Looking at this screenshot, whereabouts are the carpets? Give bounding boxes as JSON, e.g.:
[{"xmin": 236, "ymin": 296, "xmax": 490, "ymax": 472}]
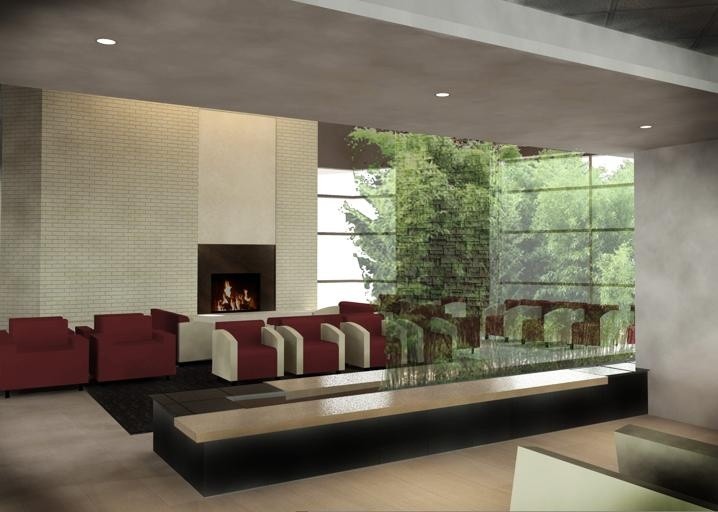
[{"xmin": 86, "ymin": 359, "xmax": 477, "ymax": 435}]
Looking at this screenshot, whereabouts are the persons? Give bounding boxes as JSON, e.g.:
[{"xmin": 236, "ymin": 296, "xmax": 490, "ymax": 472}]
[
  {"xmin": 224, "ymin": 280, "xmax": 240, "ymax": 311},
  {"xmin": 239, "ymin": 289, "xmax": 251, "ymax": 310}
]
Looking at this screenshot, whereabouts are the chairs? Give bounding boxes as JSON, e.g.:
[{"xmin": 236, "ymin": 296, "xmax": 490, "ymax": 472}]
[
  {"xmin": 574, "ymin": 303, "xmax": 620, "ymax": 348},
  {"xmin": 445, "ymin": 297, "xmax": 480, "ymax": 354},
  {"xmin": 617, "ymin": 302, "xmax": 636, "ymax": 346},
  {"xmin": 267, "ymin": 316, "xmax": 347, "ymax": 374},
  {"xmin": 335, "ymin": 313, "xmax": 406, "ymax": 369},
  {"xmin": 211, "ymin": 322, "xmax": 285, "ymax": 381},
  {"xmin": 149, "ymin": 309, "xmax": 215, "ymax": 364},
  {"xmin": 483, "ymin": 296, "xmax": 538, "ymax": 343},
  {"xmin": 0, "ymin": 314, "xmax": 176, "ymax": 399},
  {"xmin": 398, "ymin": 308, "xmax": 455, "ymax": 365},
  {"xmin": 517, "ymin": 297, "xmax": 568, "ymax": 348}
]
